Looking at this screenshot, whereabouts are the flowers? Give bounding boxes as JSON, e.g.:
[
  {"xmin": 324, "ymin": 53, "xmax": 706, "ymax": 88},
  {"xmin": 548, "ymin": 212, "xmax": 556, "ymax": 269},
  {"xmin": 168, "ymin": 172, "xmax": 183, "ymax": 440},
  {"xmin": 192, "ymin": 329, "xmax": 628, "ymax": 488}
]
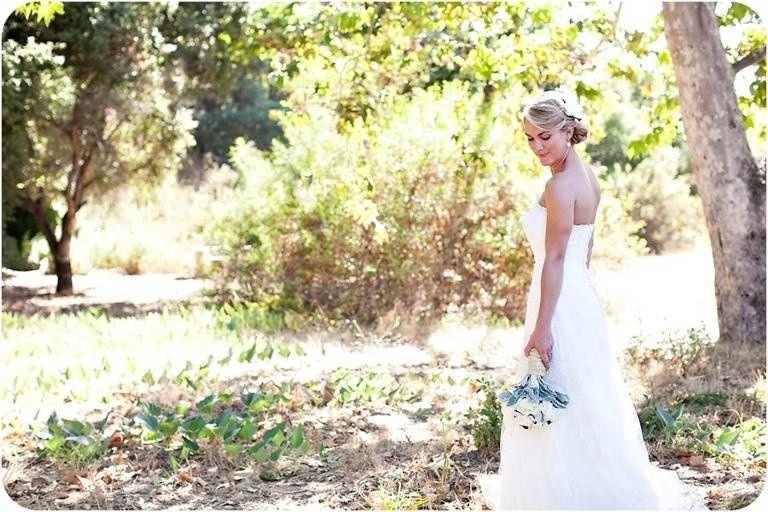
[{"xmin": 497, "ymin": 348, "xmax": 570, "ymax": 433}]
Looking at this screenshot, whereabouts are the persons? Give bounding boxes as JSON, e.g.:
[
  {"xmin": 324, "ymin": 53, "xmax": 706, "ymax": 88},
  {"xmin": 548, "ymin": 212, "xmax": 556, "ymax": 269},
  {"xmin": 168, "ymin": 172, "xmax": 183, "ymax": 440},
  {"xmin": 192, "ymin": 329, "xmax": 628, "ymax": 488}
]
[{"xmin": 475, "ymin": 89, "xmax": 707, "ymax": 512}]
